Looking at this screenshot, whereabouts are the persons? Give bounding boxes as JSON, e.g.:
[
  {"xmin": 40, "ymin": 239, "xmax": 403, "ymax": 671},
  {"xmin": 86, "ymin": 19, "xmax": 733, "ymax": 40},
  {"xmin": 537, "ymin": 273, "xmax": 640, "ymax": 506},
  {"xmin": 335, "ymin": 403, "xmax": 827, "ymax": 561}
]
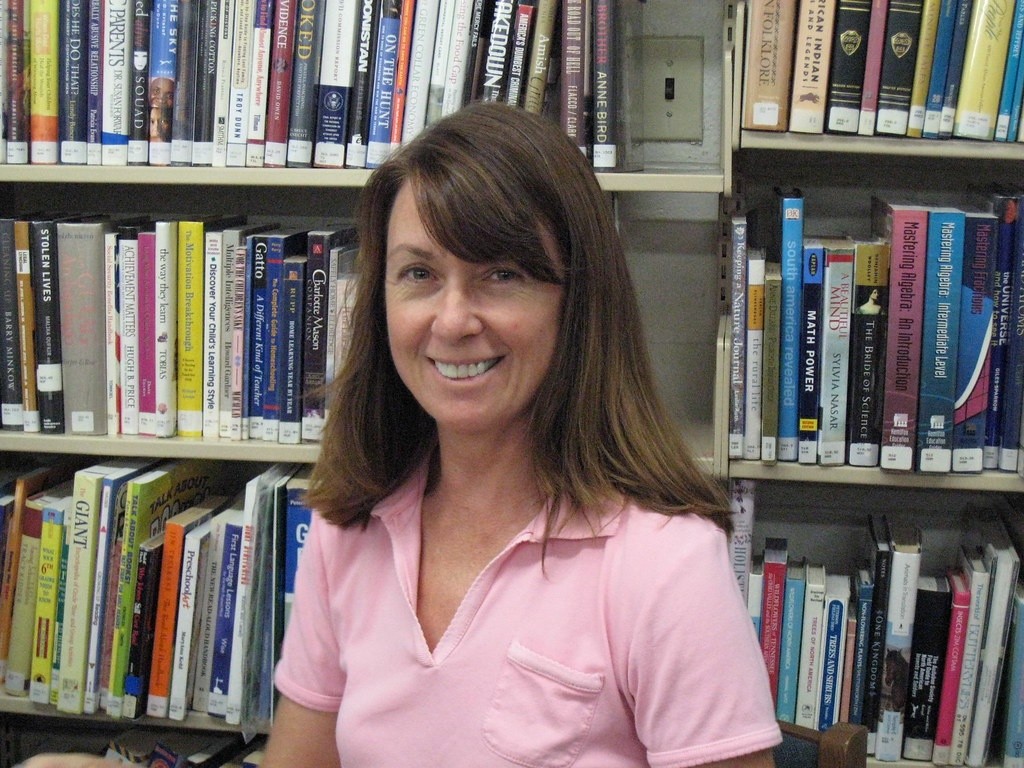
[{"xmin": 257, "ymin": 102, "xmax": 786, "ymax": 767}]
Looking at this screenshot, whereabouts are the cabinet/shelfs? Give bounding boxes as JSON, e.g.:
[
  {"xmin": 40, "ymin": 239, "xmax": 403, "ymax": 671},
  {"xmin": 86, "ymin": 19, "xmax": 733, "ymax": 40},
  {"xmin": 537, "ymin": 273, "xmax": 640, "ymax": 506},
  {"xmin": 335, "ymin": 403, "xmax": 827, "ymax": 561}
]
[
  {"xmin": 721, "ymin": 0, "xmax": 1024, "ymax": 768},
  {"xmin": 0, "ymin": 0, "xmax": 736, "ymax": 768}
]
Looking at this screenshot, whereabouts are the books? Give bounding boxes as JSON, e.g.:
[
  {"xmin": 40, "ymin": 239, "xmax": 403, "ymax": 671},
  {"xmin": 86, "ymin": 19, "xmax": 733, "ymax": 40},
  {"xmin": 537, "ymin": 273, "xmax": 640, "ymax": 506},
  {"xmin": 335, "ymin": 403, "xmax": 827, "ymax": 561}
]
[
  {"xmin": 741, "ymin": 1, "xmax": 1024, "ymax": 143},
  {"xmin": 727, "ymin": 182, "xmax": 1024, "ymax": 479},
  {"xmin": 2, "ymin": 457, "xmax": 315, "ymax": 768},
  {"xmin": 726, "ymin": 476, "xmax": 1023, "ymax": 767},
  {"xmin": 0, "ymin": 209, "xmax": 362, "ymax": 444},
  {"xmin": 1, "ymin": 0, "xmax": 646, "ymax": 174}
]
